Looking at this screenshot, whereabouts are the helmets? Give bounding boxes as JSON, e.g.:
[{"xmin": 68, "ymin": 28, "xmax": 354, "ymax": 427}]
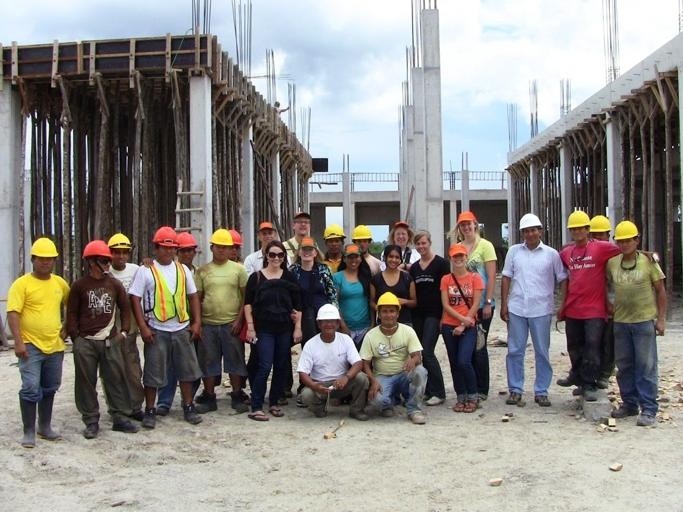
[
  {"xmin": 316, "ymin": 303, "xmax": 342, "ymax": 321},
  {"xmin": 518, "ymin": 210, "xmax": 638, "ymax": 240},
  {"xmin": 29, "ymin": 225, "xmax": 241, "ymax": 258},
  {"xmin": 375, "ymin": 291, "xmax": 402, "ymax": 312}
]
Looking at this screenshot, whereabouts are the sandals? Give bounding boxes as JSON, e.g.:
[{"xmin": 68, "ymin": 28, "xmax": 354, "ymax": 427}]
[{"xmin": 454, "ymin": 397, "xmax": 479, "ymax": 412}]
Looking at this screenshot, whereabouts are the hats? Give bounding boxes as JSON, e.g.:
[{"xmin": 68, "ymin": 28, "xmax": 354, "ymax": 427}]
[{"xmin": 257, "ymin": 211, "xmax": 477, "ymax": 257}]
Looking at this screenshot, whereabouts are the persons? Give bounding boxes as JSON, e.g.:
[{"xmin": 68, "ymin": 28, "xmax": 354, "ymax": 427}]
[
  {"xmin": 439, "ymin": 243, "xmax": 484, "ymax": 413},
  {"xmin": 289, "ymin": 222, "xmax": 451, "ymax": 405},
  {"xmin": 66, "ymin": 227, "xmax": 250, "ymax": 438},
  {"xmin": 7, "ymin": 237, "xmax": 70, "ymax": 448},
  {"xmin": 455, "ymin": 211, "xmax": 498, "ymax": 401},
  {"xmin": 244, "ymin": 239, "xmax": 302, "ymax": 423},
  {"xmin": 297, "ymin": 303, "xmax": 368, "ymax": 422},
  {"xmin": 498, "ymin": 213, "xmax": 569, "ymax": 407},
  {"xmin": 558, "ymin": 211, "xmax": 666, "ymax": 426},
  {"xmin": 359, "ymin": 292, "xmax": 427, "ymax": 423},
  {"xmin": 245, "ymin": 211, "xmax": 321, "ymax": 404}
]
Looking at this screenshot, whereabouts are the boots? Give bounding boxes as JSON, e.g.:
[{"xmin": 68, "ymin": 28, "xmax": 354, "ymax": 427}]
[
  {"xmin": 19, "ymin": 397, "xmax": 36, "ymax": 448},
  {"xmin": 37, "ymin": 392, "xmax": 62, "ymax": 441}
]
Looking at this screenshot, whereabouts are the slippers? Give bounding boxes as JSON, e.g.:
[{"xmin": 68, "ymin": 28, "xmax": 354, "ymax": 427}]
[
  {"xmin": 249, "ymin": 413, "xmax": 269, "ymax": 421},
  {"xmin": 269, "ymin": 409, "xmax": 284, "ymax": 417}
]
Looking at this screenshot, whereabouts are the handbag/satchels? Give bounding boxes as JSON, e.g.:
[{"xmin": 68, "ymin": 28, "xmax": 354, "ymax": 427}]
[
  {"xmin": 239, "ymin": 318, "xmax": 255, "ymax": 343},
  {"xmin": 475, "ymin": 324, "xmax": 487, "ymax": 351}
]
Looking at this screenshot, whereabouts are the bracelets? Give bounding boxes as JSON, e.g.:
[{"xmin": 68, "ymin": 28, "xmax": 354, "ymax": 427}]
[
  {"xmin": 247, "ymin": 329, "xmax": 255, "ymax": 332},
  {"xmin": 247, "ymin": 321, "xmax": 254, "ymax": 325},
  {"xmin": 461, "ymin": 322, "xmax": 465, "ymax": 326}
]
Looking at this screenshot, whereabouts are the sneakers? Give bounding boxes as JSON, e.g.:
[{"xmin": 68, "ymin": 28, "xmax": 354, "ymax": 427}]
[
  {"xmin": 84, "ymin": 390, "xmax": 251, "ymax": 438},
  {"xmin": 507, "ymin": 378, "xmax": 655, "ymax": 426},
  {"xmin": 277, "ymin": 389, "xmax": 445, "ymax": 424}
]
